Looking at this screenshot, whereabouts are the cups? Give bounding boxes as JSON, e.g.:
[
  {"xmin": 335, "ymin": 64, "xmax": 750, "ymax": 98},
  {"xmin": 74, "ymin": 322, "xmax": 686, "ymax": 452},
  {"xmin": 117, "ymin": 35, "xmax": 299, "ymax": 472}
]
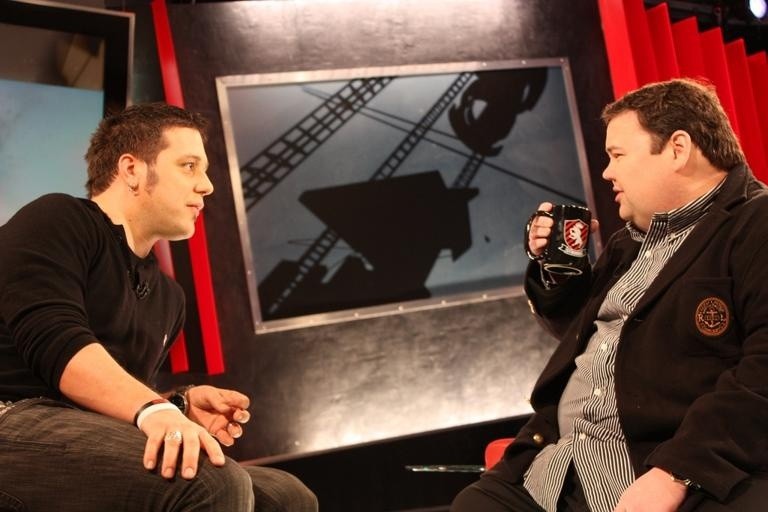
[{"xmin": 523, "ymin": 204, "xmax": 592, "ymax": 277}]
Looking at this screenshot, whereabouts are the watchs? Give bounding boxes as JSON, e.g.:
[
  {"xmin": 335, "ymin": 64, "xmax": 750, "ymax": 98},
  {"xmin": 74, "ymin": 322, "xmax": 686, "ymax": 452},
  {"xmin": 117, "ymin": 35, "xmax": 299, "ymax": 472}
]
[{"xmin": 167, "ymin": 384, "xmax": 195, "ymax": 414}]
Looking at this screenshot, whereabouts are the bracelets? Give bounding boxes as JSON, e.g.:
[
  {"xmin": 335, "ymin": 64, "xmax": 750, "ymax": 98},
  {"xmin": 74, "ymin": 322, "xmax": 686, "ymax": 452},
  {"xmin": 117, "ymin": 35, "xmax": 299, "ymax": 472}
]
[
  {"xmin": 671, "ymin": 472, "xmax": 701, "ymax": 490},
  {"xmin": 133, "ymin": 399, "xmax": 179, "ymax": 430}
]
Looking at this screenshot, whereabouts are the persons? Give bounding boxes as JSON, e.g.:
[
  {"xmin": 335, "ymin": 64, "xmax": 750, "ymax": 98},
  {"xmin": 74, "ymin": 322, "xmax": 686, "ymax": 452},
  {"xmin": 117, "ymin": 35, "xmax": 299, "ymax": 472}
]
[
  {"xmin": 451, "ymin": 79, "xmax": 768, "ymax": 512},
  {"xmin": 0, "ymin": 103, "xmax": 319, "ymax": 512}
]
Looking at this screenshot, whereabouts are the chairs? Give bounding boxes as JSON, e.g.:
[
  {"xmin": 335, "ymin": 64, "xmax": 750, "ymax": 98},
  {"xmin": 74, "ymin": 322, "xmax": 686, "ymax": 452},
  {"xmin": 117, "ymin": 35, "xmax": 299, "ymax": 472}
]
[{"xmin": 406, "ymin": 436, "xmax": 516, "ymax": 477}]
